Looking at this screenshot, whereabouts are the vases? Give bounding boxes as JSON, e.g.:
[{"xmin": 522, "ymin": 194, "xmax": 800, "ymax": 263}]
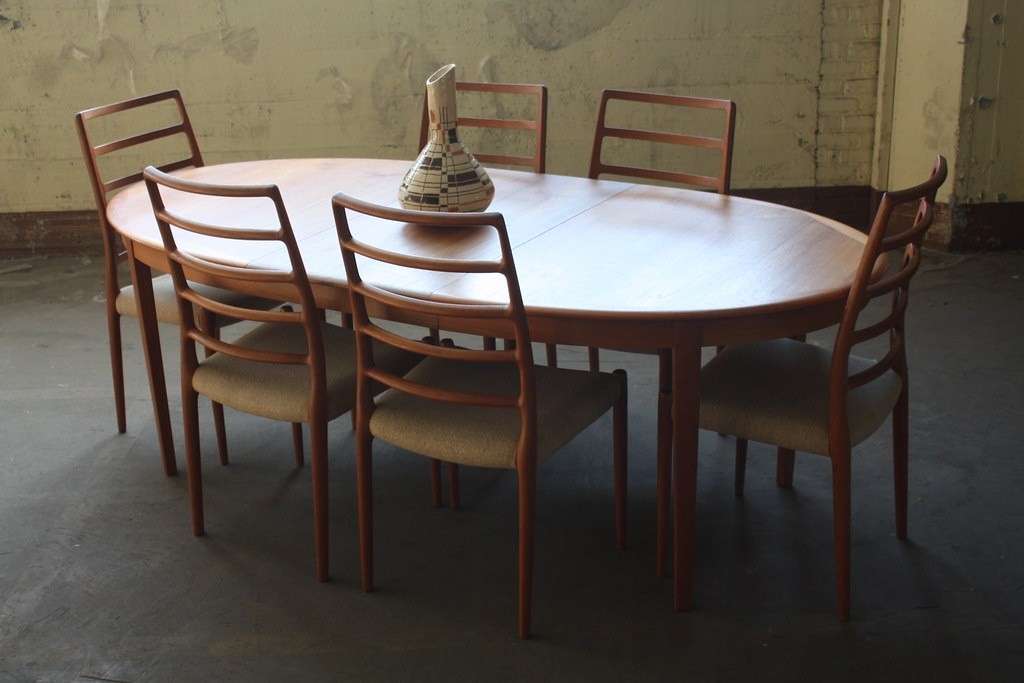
[{"xmin": 399, "ymin": 64, "xmax": 497, "ymax": 213}]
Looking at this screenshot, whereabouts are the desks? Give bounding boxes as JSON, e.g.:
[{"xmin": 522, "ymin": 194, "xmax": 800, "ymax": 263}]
[{"xmin": 106, "ymin": 158, "xmax": 886, "ymax": 616}]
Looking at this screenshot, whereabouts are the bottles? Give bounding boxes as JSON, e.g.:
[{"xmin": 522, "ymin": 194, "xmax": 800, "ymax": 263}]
[{"xmin": 398, "ymin": 64, "xmax": 494, "ymax": 212}]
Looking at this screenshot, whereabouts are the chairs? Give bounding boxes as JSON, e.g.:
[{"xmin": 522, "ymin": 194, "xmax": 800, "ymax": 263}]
[
  {"xmin": 74, "ymin": 89, "xmax": 305, "ymax": 478},
  {"xmin": 418, "ymin": 80, "xmax": 561, "ymax": 365},
  {"xmin": 544, "ymin": 88, "xmax": 737, "ymax": 439},
  {"xmin": 329, "ymin": 190, "xmax": 624, "ymax": 638},
  {"xmin": 695, "ymin": 154, "xmax": 944, "ymax": 615},
  {"xmin": 145, "ymin": 165, "xmax": 439, "ymax": 581}
]
